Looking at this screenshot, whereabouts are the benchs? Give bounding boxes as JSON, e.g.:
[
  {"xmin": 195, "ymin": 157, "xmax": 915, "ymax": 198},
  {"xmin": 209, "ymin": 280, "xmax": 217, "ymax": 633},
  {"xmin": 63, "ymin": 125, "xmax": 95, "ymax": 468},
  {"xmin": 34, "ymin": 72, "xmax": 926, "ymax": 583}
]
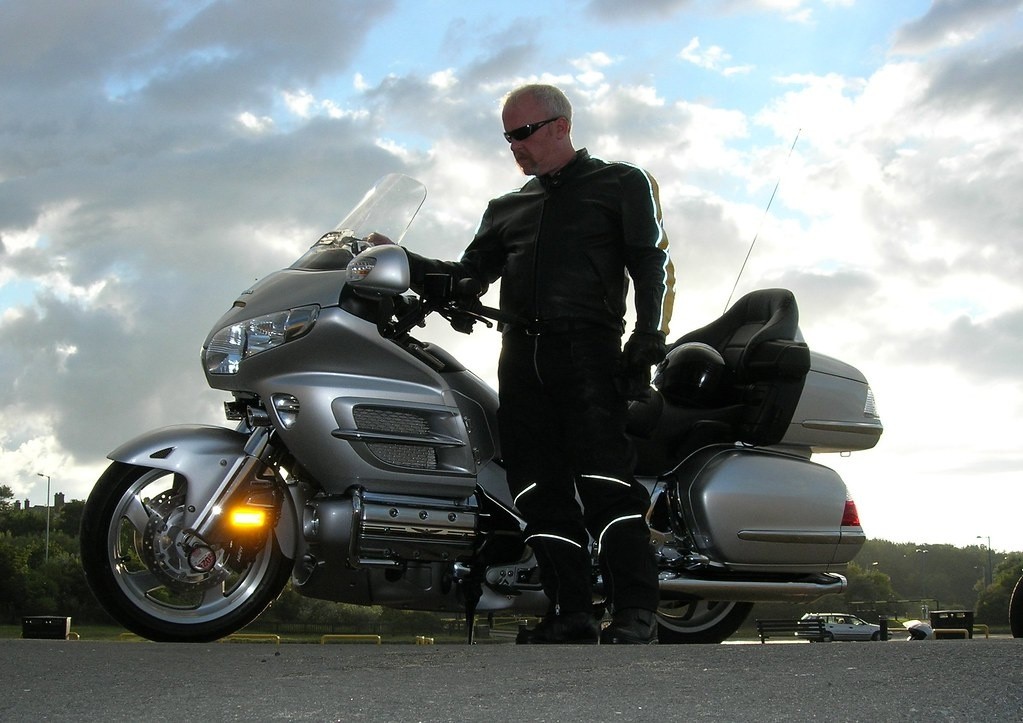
[{"xmin": 756, "ymin": 617, "xmax": 827, "ymax": 644}]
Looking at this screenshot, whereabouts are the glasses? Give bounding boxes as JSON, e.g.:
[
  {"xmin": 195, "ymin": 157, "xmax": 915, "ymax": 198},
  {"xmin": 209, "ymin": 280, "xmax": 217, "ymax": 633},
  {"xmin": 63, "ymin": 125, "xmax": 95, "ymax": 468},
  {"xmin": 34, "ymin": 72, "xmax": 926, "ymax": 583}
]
[{"xmin": 504, "ymin": 115, "xmax": 569, "ymax": 143}]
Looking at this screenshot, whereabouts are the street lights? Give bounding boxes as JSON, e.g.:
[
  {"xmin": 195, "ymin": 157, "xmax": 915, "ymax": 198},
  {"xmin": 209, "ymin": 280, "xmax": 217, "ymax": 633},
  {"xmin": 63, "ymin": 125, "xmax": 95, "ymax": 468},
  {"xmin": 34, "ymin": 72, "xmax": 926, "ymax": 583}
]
[
  {"xmin": 915, "ymin": 549, "xmax": 929, "ymax": 621},
  {"xmin": 37, "ymin": 472, "xmax": 52, "ymax": 565},
  {"xmin": 976, "ymin": 536, "xmax": 993, "ymax": 586}
]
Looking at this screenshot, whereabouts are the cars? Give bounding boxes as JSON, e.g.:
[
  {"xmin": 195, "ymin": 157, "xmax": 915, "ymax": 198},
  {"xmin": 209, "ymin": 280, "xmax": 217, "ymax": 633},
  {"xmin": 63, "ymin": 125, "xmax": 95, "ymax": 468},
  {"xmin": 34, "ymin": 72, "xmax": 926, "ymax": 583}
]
[{"xmin": 794, "ymin": 612, "xmax": 894, "ymax": 643}]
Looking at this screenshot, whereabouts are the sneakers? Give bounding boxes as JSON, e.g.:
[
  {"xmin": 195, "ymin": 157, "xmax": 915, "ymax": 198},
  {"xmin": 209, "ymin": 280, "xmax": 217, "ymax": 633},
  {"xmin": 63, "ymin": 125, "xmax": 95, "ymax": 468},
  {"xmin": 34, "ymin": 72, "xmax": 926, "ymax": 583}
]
[
  {"xmin": 599, "ymin": 608, "xmax": 658, "ymax": 645},
  {"xmin": 515, "ymin": 612, "xmax": 598, "ymax": 644}
]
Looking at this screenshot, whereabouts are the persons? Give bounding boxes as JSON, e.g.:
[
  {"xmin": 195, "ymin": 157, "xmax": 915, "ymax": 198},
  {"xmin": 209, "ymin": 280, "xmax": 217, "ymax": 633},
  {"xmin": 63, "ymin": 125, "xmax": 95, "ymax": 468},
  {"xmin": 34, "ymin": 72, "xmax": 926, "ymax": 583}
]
[
  {"xmin": 362, "ymin": 84, "xmax": 676, "ymax": 645},
  {"xmin": 902, "ymin": 620, "xmax": 932, "ymax": 642}
]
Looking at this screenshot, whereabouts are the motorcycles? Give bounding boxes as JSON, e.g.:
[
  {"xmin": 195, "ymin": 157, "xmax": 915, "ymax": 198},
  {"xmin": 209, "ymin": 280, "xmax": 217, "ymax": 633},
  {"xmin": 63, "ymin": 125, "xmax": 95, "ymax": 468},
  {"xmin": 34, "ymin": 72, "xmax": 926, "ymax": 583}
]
[{"xmin": 77, "ymin": 178, "xmax": 884, "ymax": 651}]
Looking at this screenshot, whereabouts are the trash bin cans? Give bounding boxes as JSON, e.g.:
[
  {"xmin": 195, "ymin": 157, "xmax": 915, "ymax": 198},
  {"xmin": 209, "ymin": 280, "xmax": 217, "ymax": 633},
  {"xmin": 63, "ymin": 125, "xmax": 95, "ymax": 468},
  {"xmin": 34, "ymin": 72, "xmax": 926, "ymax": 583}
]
[
  {"xmin": 929, "ymin": 610, "xmax": 974, "ymax": 639},
  {"xmin": 22, "ymin": 615, "xmax": 72, "ymax": 640}
]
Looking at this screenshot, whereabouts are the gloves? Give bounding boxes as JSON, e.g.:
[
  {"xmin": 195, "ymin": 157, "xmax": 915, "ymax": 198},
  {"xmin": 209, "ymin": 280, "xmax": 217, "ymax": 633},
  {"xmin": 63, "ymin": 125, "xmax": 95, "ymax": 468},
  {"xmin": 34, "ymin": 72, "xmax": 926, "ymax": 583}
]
[{"xmin": 614, "ymin": 329, "xmax": 667, "ymax": 398}]
